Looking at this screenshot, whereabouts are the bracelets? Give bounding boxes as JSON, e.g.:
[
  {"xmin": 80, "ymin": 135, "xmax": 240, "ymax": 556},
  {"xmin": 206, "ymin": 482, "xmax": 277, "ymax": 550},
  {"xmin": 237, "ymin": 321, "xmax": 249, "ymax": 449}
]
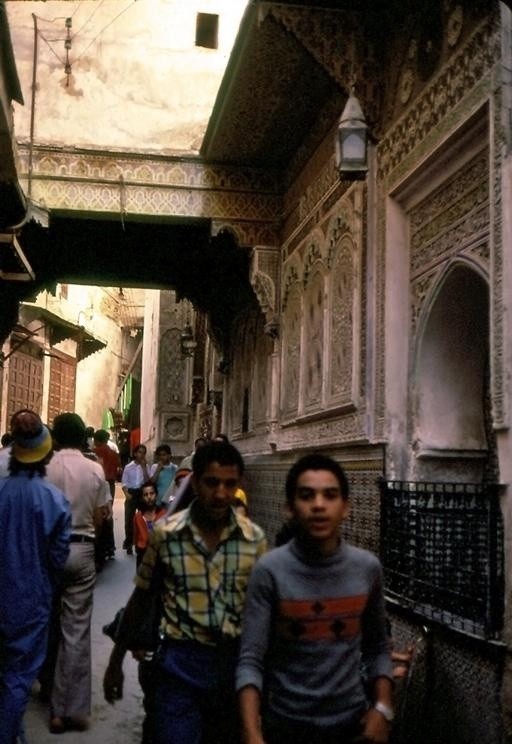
[{"xmin": 374, "ymin": 701, "xmax": 394, "ymax": 721}]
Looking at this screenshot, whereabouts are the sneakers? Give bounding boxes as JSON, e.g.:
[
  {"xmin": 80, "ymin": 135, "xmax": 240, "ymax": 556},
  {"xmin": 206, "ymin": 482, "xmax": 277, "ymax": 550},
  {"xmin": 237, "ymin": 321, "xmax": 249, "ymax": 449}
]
[{"xmin": 48, "ymin": 714, "xmax": 89, "ymax": 734}]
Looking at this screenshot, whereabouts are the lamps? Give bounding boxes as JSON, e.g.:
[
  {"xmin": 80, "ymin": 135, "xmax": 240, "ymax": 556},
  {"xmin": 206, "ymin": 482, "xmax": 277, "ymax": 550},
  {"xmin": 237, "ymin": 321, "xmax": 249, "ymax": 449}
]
[
  {"xmin": 333, "ymin": 57, "xmax": 370, "ymax": 181},
  {"xmin": 179, "ymin": 299, "xmax": 198, "ymax": 358}
]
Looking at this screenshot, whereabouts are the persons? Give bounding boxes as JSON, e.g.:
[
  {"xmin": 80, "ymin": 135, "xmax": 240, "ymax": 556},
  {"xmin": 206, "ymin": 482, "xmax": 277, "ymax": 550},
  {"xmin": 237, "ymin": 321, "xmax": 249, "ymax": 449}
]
[
  {"xmin": 1, "ymin": 406, "xmax": 252, "ymax": 577},
  {"xmin": 1, "ymin": 412, "xmax": 74, "ymax": 742},
  {"xmin": 236, "ymin": 446, "xmax": 398, "ymax": 743},
  {"xmin": 105, "ymin": 440, "xmax": 276, "ymax": 744},
  {"xmin": 42, "ymin": 411, "xmax": 111, "ymax": 732}
]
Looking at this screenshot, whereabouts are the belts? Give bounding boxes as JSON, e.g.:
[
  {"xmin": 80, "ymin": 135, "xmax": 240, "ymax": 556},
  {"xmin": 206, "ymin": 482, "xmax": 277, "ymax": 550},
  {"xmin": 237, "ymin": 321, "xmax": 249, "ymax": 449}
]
[{"xmin": 67, "ymin": 535, "xmax": 94, "ymax": 543}]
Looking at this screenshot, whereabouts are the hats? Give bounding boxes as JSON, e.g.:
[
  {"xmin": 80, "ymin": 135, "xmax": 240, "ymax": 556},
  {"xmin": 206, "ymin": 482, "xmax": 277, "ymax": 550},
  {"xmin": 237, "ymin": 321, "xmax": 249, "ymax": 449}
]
[{"xmin": 10, "ymin": 409, "xmax": 54, "ymax": 464}]
